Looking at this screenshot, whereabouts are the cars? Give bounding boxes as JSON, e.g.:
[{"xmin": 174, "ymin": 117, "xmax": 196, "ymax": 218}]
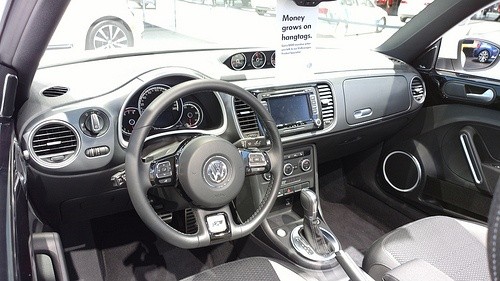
[
  {"xmin": 43, "ymin": 0, "xmax": 143, "ymax": 53},
  {"xmin": 472, "ymin": 41, "xmax": 499, "ymax": 63},
  {"xmin": 397, "ymin": 0, "xmax": 434, "ymax": 22},
  {"xmin": 250, "ymin": 0, "xmax": 276, "ymax": 15},
  {"xmin": 314, "ymin": 0, "xmax": 388, "ymax": 33}
]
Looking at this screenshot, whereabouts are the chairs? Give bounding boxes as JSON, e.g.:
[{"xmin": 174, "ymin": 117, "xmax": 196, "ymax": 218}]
[
  {"xmin": 360, "ymin": 174, "xmax": 500, "ymax": 281},
  {"xmin": 170, "ymin": 253, "xmax": 299, "ymax": 281}
]
